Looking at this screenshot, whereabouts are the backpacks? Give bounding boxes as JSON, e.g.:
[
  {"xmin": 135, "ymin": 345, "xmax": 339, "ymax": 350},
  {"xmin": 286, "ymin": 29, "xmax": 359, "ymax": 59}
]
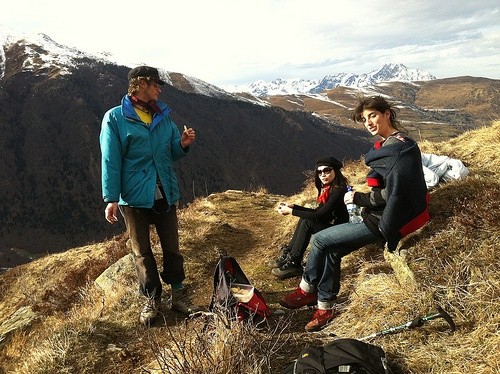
[
  {"xmin": 284, "ymin": 339, "xmax": 404, "ymax": 374},
  {"xmin": 202, "ymin": 248, "xmax": 271, "ymax": 332}
]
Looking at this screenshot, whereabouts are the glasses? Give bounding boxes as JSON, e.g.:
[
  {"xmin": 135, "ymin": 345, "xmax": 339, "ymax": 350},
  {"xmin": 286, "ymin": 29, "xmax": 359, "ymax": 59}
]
[{"xmin": 316, "ymin": 167, "xmax": 333, "ymax": 176}]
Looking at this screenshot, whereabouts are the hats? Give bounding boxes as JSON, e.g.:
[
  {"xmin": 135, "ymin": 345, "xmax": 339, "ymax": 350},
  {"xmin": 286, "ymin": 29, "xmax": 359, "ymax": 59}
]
[
  {"xmin": 316, "ymin": 156, "xmax": 343, "ymax": 170},
  {"xmin": 127, "ymin": 64, "xmax": 165, "ymax": 84}
]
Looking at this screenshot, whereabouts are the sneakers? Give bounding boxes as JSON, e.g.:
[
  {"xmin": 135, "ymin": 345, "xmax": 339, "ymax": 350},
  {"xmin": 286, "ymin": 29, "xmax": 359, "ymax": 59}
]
[
  {"xmin": 278, "ymin": 287, "xmax": 318, "ymax": 309},
  {"xmin": 172, "ymin": 286, "xmax": 200, "ymax": 316},
  {"xmin": 140, "ymin": 287, "xmax": 162, "ymax": 325},
  {"xmin": 271, "ymin": 252, "xmax": 303, "ymax": 279},
  {"xmin": 269, "ymin": 248, "xmax": 290, "ymax": 268},
  {"xmin": 305, "ymin": 309, "xmax": 335, "ymax": 331}
]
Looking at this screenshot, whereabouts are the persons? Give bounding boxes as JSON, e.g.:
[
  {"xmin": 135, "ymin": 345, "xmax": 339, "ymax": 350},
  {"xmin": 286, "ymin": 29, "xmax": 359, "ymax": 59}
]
[
  {"xmin": 279, "ymin": 96, "xmax": 431, "ymax": 332},
  {"xmin": 99, "ymin": 67, "xmax": 196, "ymax": 326},
  {"xmin": 268, "ymin": 156, "xmax": 350, "ymax": 279}
]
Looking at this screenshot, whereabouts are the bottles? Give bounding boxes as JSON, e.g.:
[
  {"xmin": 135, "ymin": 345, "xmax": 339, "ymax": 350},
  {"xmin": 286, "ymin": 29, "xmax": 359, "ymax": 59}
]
[{"xmin": 346, "ymin": 186, "xmax": 363, "ymax": 225}]
